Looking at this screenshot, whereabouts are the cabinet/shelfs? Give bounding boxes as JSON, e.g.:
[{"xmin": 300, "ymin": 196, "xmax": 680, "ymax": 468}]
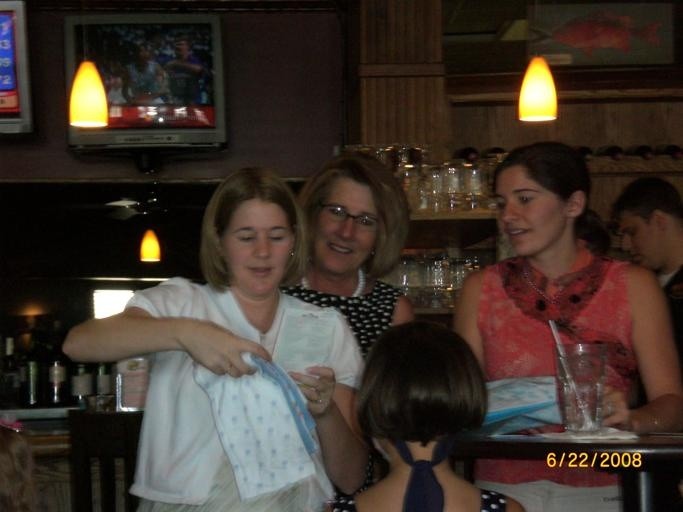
[{"xmin": 332, "ymin": 143, "xmax": 513, "ymax": 315}]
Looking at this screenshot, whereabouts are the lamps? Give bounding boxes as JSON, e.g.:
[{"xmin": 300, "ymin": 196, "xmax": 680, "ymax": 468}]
[
  {"xmin": 139, "ymin": 229, "xmax": 161, "ymax": 262},
  {"xmin": 69, "ymin": 2, "xmax": 108, "ymax": 130},
  {"xmin": 7, "ymin": 276, "xmax": 54, "ymax": 349},
  {"xmin": 518, "ymin": 3, "xmax": 558, "ymax": 123}
]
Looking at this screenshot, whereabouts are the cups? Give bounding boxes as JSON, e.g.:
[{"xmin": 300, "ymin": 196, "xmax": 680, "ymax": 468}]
[
  {"xmin": 413, "ymin": 166, "xmax": 488, "ymax": 211},
  {"xmin": 396, "ymin": 258, "xmax": 469, "ymax": 309},
  {"xmin": 554, "ymin": 342, "xmax": 609, "ymax": 438}
]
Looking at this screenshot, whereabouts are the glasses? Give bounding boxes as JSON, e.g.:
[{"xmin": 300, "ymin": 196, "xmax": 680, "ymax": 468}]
[{"xmin": 316, "ymin": 196, "xmax": 380, "ymax": 233}]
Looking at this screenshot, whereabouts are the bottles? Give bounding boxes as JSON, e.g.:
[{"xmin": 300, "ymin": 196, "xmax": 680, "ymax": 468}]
[
  {"xmin": 48, "ymin": 321, "xmax": 70, "ymax": 407},
  {"xmin": 93, "ymin": 363, "xmax": 112, "ymax": 397},
  {"xmin": 27, "ymin": 330, "xmax": 42, "ymax": 409},
  {"xmin": 72, "ymin": 362, "xmax": 92, "ymax": 406},
  {"xmin": 1, "ymin": 337, "xmax": 22, "ymax": 409}
]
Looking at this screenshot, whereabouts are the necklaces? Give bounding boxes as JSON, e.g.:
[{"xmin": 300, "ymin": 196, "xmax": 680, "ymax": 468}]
[
  {"xmin": 299, "ymin": 258, "xmax": 365, "ymax": 298},
  {"xmin": 521, "ymin": 256, "xmax": 580, "ymax": 304}
]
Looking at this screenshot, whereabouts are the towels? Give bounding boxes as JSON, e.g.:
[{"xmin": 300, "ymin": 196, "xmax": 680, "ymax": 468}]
[
  {"xmin": 540, "ymin": 423, "xmax": 637, "ymax": 440},
  {"xmin": 193, "ymin": 351, "xmax": 319, "ymax": 500},
  {"xmin": 482, "ymin": 374, "xmax": 602, "ymax": 437}
]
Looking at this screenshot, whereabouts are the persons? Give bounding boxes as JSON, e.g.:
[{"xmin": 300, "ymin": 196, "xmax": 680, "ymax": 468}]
[
  {"xmin": 450, "ymin": 140, "xmax": 682, "ymax": 512},
  {"xmin": 610, "ymin": 176, "xmax": 683, "ymax": 364},
  {"xmin": 62, "ymin": 165, "xmax": 365, "ymax": 511},
  {"xmin": 277, "ymin": 150, "xmax": 415, "ymax": 365},
  {"xmin": 164, "ymin": 38, "xmax": 209, "ymax": 106},
  {"xmin": 118, "ymin": 40, "xmax": 167, "ymax": 105},
  {"xmin": 326, "ymin": 321, "xmax": 526, "ymax": 511}
]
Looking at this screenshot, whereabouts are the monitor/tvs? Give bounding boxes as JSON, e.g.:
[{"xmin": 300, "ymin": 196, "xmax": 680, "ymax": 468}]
[
  {"xmin": 62, "ymin": 12, "xmax": 227, "ymax": 152},
  {"xmin": 0, "ymin": 0, "xmax": 33, "ymax": 135}
]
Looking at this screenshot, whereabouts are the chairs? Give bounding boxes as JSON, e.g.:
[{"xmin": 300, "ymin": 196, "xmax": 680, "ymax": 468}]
[{"xmin": 65, "ymin": 410, "xmax": 143, "ymax": 512}]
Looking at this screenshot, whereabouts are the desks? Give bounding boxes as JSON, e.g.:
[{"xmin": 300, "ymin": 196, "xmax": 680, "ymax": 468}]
[{"xmin": 1, "ymin": 418, "xmax": 141, "ymax": 512}]
